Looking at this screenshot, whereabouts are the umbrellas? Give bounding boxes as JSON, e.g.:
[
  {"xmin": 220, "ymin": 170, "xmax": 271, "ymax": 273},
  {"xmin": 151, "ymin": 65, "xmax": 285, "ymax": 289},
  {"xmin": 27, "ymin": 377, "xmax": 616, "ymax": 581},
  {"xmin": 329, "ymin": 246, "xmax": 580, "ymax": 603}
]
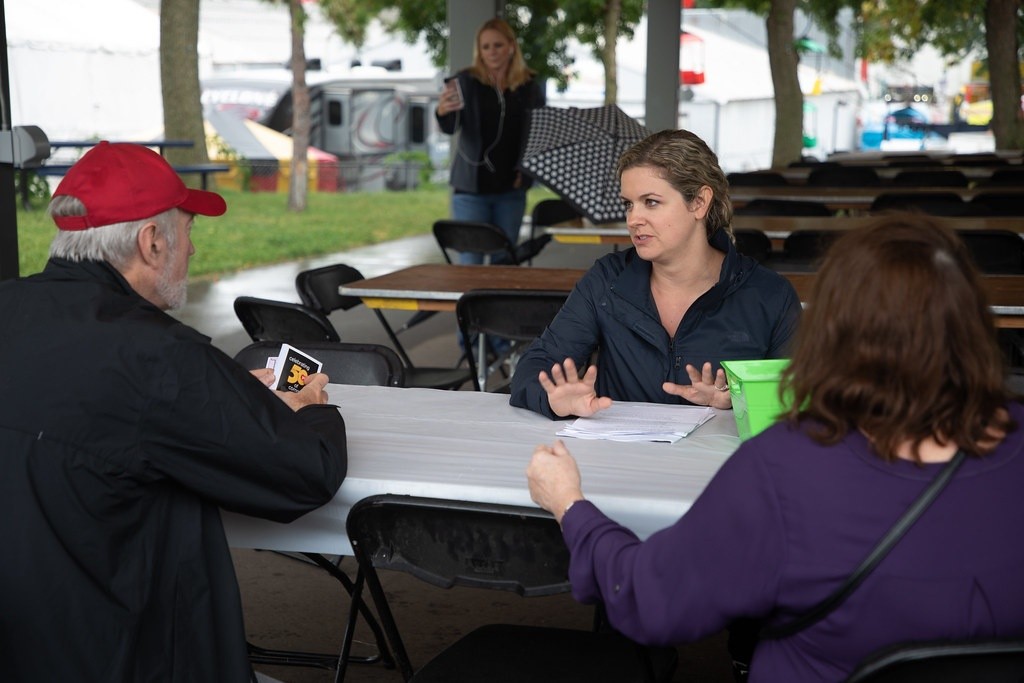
[{"xmin": 520, "ymin": 95, "xmax": 654, "ymax": 227}]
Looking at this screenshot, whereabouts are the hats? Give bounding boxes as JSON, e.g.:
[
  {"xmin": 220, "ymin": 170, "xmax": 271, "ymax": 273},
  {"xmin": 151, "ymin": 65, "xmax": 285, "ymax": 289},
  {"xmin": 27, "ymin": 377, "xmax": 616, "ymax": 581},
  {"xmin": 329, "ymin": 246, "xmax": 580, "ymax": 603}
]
[{"xmin": 51, "ymin": 141, "xmax": 226, "ymax": 230}]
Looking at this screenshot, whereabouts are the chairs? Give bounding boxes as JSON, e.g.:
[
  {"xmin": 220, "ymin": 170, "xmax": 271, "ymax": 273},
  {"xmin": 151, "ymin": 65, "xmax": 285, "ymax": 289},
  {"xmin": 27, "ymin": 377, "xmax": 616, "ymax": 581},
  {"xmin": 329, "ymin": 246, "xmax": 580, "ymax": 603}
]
[
  {"xmin": 783, "ymin": 230, "xmax": 832, "ymax": 257},
  {"xmin": 456, "ymin": 287, "xmax": 572, "ymax": 391},
  {"xmin": 530, "ymin": 200, "xmax": 581, "ymax": 266},
  {"xmin": 729, "ymin": 154, "xmax": 1024, "ymax": 217},
  {"xmin": 433, "ymin": 220, "xmax": 520, "ymax": 265},
  {"xmin": 296, "ymin": 264, "xmax": 473, "ymax": 390},
  {"xmin": 233, "ymin": 341, "xmax": 405, "ymax": 387},
  {"xmin": 234, "ymin": 296, "xmax": 342, "ymax": 342},
  {"xmin": 955, "ymin": 228, "xmax": 1024, "ymax": 274},
  {"xmin": 734, "ymin": 228, "xmax": 772, "ymax": 255},
  {"xmin": 332, "ymin": 493, "xmax": 680, "ymax": 683}
]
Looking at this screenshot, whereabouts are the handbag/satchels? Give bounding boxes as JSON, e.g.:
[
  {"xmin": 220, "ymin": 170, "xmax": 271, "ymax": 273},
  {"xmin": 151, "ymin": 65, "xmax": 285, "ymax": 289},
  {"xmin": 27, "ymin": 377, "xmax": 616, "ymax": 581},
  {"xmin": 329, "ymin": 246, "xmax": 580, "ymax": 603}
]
[{"xmin": 727, "ymin": 617, "xmax": 760, "ymax": 683}]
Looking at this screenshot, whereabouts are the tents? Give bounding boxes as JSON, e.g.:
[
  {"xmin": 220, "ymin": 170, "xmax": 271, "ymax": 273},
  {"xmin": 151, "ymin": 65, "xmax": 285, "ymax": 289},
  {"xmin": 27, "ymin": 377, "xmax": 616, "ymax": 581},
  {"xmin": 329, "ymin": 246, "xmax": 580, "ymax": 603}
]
[
  {"xmin": 163, "ymin": 115, "xmax": 341, "ymax": 196},
  {"xmin": 198, "ymin": 13, "xmax": 862, "ymax": 178}
]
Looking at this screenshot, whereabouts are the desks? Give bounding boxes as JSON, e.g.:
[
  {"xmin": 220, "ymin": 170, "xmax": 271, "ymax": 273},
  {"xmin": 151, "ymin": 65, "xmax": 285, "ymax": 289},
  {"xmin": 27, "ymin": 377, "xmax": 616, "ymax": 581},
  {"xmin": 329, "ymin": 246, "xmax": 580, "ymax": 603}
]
[
  {"xmin": 339, "ymin": 264, "xmax": 1024, "ymax": 367},
  {"xmin": 544, "ymin": 216, "xmax": 1024, "ymax": 253},
  {"xmin": 18, "ymin": 140, "xmax": 195, "ymax": 211},
  {"xmin": 219, "ymin": 383, "xmax": 741, "ymax": 556},
  {"xmin": 727, "ymin": 187, "xmax": 1024, "ymax": 216},
  {"xmin": 768, "ymin": 168, "xmax": 1024, "ymax": 184}
]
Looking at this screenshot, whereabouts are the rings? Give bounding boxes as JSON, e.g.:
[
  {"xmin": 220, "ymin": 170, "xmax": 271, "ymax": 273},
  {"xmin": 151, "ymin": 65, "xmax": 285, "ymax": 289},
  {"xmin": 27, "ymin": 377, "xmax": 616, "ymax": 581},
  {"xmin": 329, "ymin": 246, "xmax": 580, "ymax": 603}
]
[{"xmin": 715, "ymin": 381, "xmax": 729, "ymax": 391}]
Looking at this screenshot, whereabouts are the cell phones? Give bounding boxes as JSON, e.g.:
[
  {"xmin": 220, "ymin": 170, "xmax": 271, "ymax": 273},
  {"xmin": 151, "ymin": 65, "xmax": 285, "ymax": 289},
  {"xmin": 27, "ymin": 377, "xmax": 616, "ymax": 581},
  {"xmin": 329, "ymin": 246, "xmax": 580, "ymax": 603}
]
[{"xmin": 447, "ymin": 79, "xmax": 464, "ymax": 107}]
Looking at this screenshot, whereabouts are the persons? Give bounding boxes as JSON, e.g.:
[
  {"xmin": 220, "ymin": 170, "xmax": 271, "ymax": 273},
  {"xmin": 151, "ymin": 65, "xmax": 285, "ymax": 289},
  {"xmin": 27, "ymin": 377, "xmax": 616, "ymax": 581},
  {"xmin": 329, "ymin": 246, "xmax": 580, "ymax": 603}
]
[
  {"xmin": 0, "ymin": 138, "xmax": 348, "ymax": 683},
  {"xmin": 528, "ymin": 216, "xmax": 1023, "ymax": 683},
  {"xmin": 510, "ymin": 127, "xmax": 804, "ymax": 425},
  {"xmin": 434, "ymin": 15, "xmax": 545, "ymax": 379}
]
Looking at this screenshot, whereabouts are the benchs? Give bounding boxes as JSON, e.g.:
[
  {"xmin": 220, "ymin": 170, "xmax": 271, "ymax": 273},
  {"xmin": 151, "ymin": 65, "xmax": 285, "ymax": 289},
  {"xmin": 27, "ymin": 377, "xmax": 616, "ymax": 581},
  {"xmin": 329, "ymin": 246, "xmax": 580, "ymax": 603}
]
[{"xmin": 36, "ymin": 162, "xmax": 231, "ymax": 191}]
